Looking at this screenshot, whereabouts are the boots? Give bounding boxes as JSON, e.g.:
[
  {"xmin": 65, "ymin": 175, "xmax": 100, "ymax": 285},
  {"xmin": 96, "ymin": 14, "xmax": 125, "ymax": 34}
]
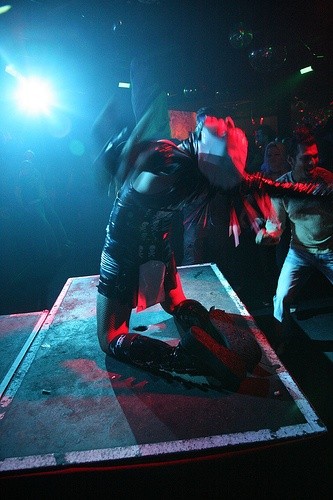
[
  {"xmin": 177, "ymin": 298, "xmax": 244, "ymax": 366},
  {"xmin": 108, "ymin": 326, "xmax": 240, "ymax": 390}
]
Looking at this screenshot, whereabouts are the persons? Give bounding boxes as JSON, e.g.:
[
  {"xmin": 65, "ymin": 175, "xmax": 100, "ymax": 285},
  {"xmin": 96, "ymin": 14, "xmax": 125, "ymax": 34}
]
[
  {"xmin": 254, "ymin": 129, "xmax": 333, "ymax": 370},
  {"xmin": 1, "ymin": 104, "xmax": 332, "ymax": 318},
  {"xmin": 96, "ymin": 111, "xmax": 333, "ymax": 396}
]
